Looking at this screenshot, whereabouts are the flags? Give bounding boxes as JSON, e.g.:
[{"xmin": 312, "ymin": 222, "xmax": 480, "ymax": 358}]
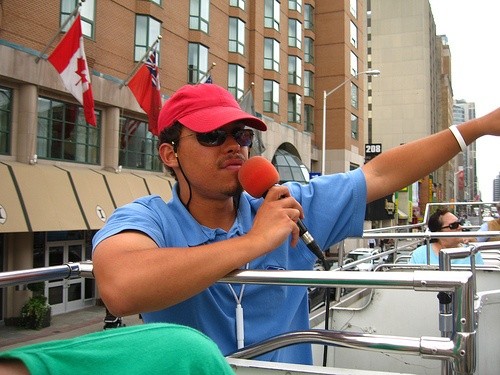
[
  {"xmin": 121, "ymin": 118, "xmax": 141, "ymax": 149},
  {"xmin": 55, "ymin": 107, "xmax": 78, "ymax": 139},
  {"xmin": 48, "ymin": 14, "xmax": 97, "ymax": 127},
  {"xmin": 128, "ymin": 48, "xmax": 162, "ymax": 136},
  {"xmin": 238, "ymin": 90, "xmax": 266, "ymax": 157}
]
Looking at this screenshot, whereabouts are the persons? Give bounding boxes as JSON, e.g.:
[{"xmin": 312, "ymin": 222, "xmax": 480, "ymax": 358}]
[
  {"xmin": 368, "ymin": 239, "xmax": 376, "ymax": 248},
  {"xmin": 463, "ymin": 203, "xmax": 500, "ymax": 243},
  {"xmin": 380, "ymin": 239, "xmax": 394, "ymax": 251},
  {"xmin": 0, "ymin": 322, "xmax": 236, "ymax": 375},
  {"xmin": 408, "ymin": 209, "xmax": 484, "ymax": 264},
  {"xmin": 90, "ymin": 85, "xmax": 500, "ymax": 365}
]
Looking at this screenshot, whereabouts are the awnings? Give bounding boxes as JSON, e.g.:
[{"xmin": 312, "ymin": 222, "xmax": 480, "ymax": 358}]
[{"xmin": 0, "ymin": 160, "xmax": 177, "ymax": 233}]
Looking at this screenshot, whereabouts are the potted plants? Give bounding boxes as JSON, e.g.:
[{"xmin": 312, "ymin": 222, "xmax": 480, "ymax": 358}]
[{"xmin": 21, "ymin": 293, "xmax": 51, "ymax": 331}]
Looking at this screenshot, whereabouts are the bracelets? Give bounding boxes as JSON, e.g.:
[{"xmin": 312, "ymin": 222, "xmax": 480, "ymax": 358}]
[{"xmin": 449, "ymin": 125, "xmax": 467, "ymax": 152}]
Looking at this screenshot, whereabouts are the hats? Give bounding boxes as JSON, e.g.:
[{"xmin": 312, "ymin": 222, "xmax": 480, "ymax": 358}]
[{"xmin": 157, "ymin": 84, "xmax": 267, "ymax": 138}]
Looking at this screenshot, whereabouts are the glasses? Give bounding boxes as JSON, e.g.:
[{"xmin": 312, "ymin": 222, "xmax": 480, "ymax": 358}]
[
  {"xmin": 440, "ymin": 222, "xmax": 461, "ymax": 230},
  {"xmin": 167, "ymin": 129, "xmax": 253, "ymax": 147}
]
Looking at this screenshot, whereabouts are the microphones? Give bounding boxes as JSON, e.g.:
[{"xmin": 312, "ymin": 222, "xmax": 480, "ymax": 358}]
[{"xmin": 238, "ymin": 156, "xmax": 326, "ymax": 260}]
